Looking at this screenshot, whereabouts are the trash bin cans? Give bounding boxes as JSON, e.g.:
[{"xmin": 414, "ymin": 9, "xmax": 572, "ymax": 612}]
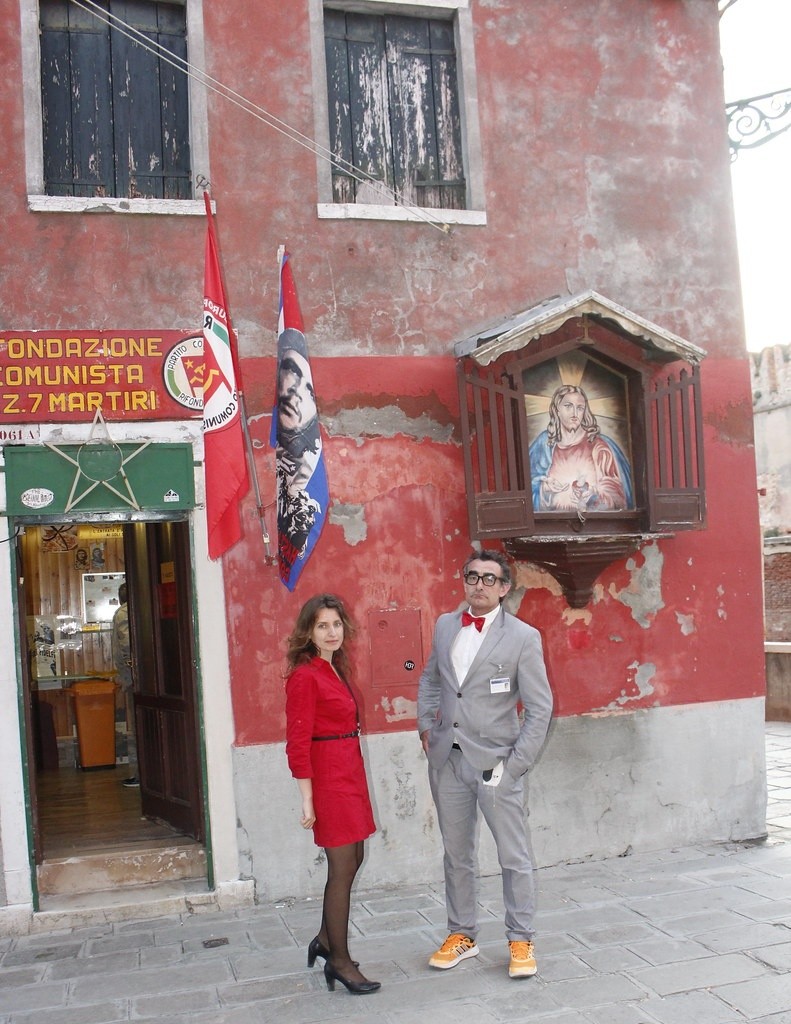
[{"xmin": 63, "ymin": 676, "xmax": 122, "ymax": 772}]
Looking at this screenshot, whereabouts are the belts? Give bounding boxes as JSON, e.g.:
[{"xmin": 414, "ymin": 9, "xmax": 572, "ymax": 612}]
[
  {"xmin": 311, "ymin": 731, "xmax": 358, "ymax": 741},
  {"xmin": 452, "ymin": 743, "xmax": 461, "ymax": 750}
]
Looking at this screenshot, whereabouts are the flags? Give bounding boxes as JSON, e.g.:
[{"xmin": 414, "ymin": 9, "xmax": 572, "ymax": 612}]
[
  {"xmin": 202, "ymin": 190, "xmax": 261, "ymax": 562},
  {"xmin": 269, "ymin": 245, "xmax": 329, "ymax": 593}
]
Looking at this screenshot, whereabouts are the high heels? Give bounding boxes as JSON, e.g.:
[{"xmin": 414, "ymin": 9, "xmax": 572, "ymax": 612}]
[
  {"xmin": 306, "ymin": 936, "xmax": 360, "ymax": 969},
  {"xmin": 323, "ymin": 958, "xmax": 382, "ymax": 994}
]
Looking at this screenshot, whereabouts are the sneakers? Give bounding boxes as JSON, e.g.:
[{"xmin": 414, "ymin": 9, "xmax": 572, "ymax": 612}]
[
  {"xmin": 507, "ymin": 939, "xmax": 537, "ymax": 978},
  {"xmin": 428, "ymin": 932, "xmax": 480, "ymax": 970}
]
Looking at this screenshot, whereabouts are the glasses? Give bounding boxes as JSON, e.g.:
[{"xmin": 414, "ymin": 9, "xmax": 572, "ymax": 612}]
[{"xmin": 463, "ymin": 573, "xmax": 504, "ymax": 587}]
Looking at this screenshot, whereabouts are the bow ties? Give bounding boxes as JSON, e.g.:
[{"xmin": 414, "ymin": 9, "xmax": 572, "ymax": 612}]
[{"xmin": 461, "ymin": 609, "xmax": 486, "ymax": 633}]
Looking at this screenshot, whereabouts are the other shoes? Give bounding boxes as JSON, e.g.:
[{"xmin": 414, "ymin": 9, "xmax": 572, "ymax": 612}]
[{"xmin": 120, "ymin": 777, "xmax": 140, "ymax": 788}]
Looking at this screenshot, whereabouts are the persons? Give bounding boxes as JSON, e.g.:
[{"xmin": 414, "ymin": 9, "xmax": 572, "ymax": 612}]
[
  {"xmin": 416, "ymin": 550, "xmax": 552, "ymax": 977},
  {"xmin": 277, "ymin": 328, "xmax": 318, "ymax": 495},
  {"xmin": 113, "ymin": 583, "xmax": 142, "ymax": 788},
  {"xmin": 284, "ymin": 594, "xmax": 381, "ymax": 994}
]
